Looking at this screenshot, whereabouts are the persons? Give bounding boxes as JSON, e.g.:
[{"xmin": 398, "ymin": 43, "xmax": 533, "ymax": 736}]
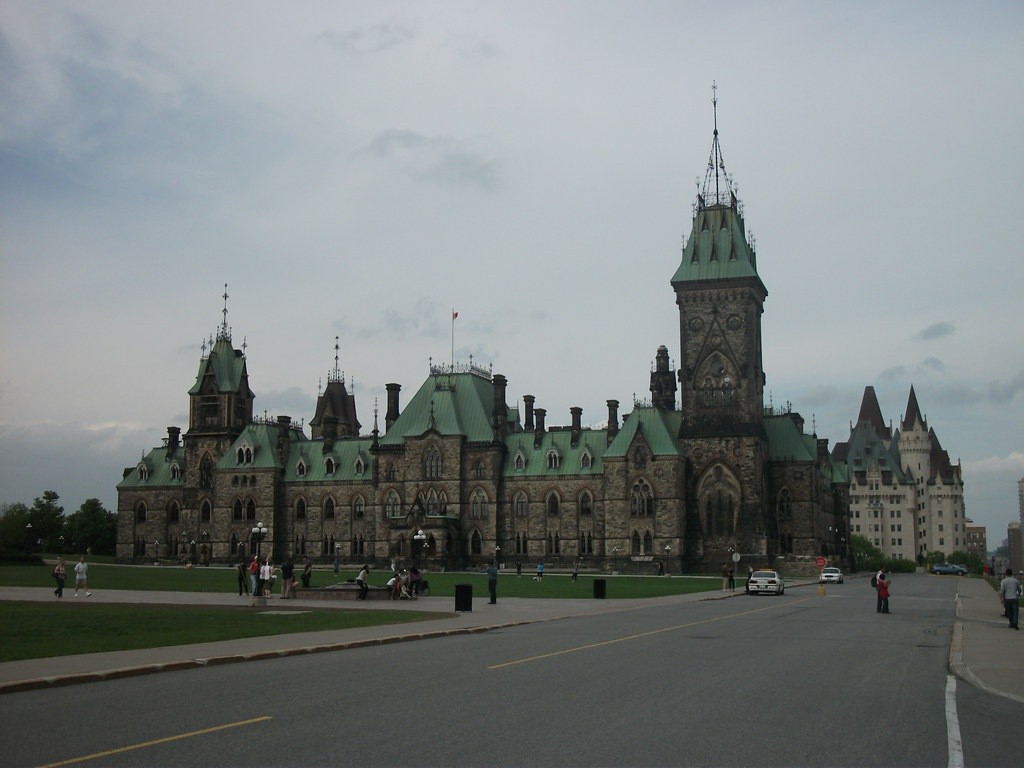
[
  {"xmin": 572, "ymin": 560, "xmax": 578, "ymax": 583},
  {"xmin": 720, "ymin": 562, "xmax": 735, "ymax": 592},
  {"xmin": 480, "ymin": 560, "xmax": 497, "ymax": 605},
  {"xmin": 53, "ymin": 560, "xmax": 67, "ymax": 599},
  {"xmin": 280, "ymin": 558, "xmax": 299, "ymax": 600},
  {"xmin": 334, "ymin": 558, "xmax": 339, "ymax": 577},
  {"xmin": 516, "ymin": 559, "xmax": 522, "ymax": 578},
  {"xmin": 658, "ymin": 559, "xmax": 665, "ymax": 576},
  {"xmin": 249, "ymin": 555, "xmax": 277, "ymax": 598},
  {"xmin": 744, "ymin": 564, "xmax": 754, "ymax": 593},
  {"xmin": 875, "ymin": 567, "xmax": 891, "ymax": 614},
  {"xmin": 983, "ymin": 563, "xmax": 989, "ymax": 578},
  {"xmin": 386, "ymin": 552, "xmax": 421, "ymax": 601},
  {"xmin": 301, "ymin": 555, "xmax": 311, "ymax": 588},
  {"xmin": 999, "ymin": 568, "xmax": 1021, "ymax": 630},
  {"xmin": 75, "ymin": 555, "xmax": 92, "ymax": 597},
  {"xmin": 355, "ymin": 564, "xmax": 369, "ymax": 601},
  {"xmin": 537, "ymin": 562, "xmax": 543, "ymax": 582},
  {"xmin": 237, "ymin": 559, "xmax": 249, "ymax": 596}
]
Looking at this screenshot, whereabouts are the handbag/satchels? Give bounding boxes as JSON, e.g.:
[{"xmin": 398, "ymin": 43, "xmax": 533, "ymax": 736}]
[
  {"xmin": 52, "ymin": 569, "xmax": 60, "ymax": 577},
  {"xmin": 269, "ymin": 575, "xmax": 277, "ymax": 584}
]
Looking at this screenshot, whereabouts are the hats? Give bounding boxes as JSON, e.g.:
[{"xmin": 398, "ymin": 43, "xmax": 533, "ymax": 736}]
[{"xmin": 255, "ymin": 556, "xmax": 258, "ymax": 559}]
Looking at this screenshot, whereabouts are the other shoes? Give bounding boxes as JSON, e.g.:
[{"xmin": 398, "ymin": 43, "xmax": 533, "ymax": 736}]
[
  {"xmin": 54, "ymin": 591, "xmax": 57, "ymax": 597},
  {"xmin": 1008, "ymin": 624, "xmax": 1015, "ymax": 628},
  {"xmin": 1001, "ymin": 614, "xmax": 1006, "ymax": 617},
  {"xmin": 86, "ymin": 593, "xmax": 92, "ymax": 597},
  {"xmin": 75, "ymin": 593, "xmax": 78, "ymax": 596},
  {"xmin": 1015, "ymin": 626, "xmax": 1019, "ymax": 630}
]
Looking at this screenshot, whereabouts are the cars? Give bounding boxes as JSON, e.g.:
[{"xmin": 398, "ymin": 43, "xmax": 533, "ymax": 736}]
[
  {"xmin": 819, "ymin": 568, "xmax": 844, "ymax": 584},
  {"xmin": 932, "ymin": 563, "xmax": 968, "ymax": 576},
  {"xmin": 748, "ymin": 571, "xmax": 785, "ymax": 596}
]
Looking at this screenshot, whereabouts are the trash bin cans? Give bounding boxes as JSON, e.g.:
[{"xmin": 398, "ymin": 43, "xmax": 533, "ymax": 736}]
[
  {"xmin": 455, "ymin": 584, "xmax": 473, "ymax": 611},
  {"xmin": 594, "ymin": 578, "xmax": 605, "ymax": 598}
]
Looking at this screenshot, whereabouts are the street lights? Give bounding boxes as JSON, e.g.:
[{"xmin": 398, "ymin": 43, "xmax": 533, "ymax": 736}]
[
  {"xmin": 413, "ymin": 530, "xmax": 426, "ymax": 569},
  {"xmin": 423, "ymin": 543, "xmax": 430, "ymax": 569},
  {"xmin": 612, "ymin": 547, "xmax": 619, "ymax": 571},
  {"xmin": 664, "ymin": 545, "xmax": 671, "ymax": 574},
  {"xmin": 495, "ymin": 546, "xmax": 501, "ymax": 570},
  {"xmin": 251, "ymin": 522, "xmax": 269, "ymax": 596},
  {"xmin": 728, "ymin": 548, "xmax": 734, "ymax": 561},
  {"xmin": 336, "ymin": 544, "xmax": 341, "ymax": 563}
]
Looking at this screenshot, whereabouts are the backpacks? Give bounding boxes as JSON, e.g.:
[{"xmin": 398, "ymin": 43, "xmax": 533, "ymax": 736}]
[{"xmin": 871, "ymin": 574, "xmax": 877, "ymax": 587}]
